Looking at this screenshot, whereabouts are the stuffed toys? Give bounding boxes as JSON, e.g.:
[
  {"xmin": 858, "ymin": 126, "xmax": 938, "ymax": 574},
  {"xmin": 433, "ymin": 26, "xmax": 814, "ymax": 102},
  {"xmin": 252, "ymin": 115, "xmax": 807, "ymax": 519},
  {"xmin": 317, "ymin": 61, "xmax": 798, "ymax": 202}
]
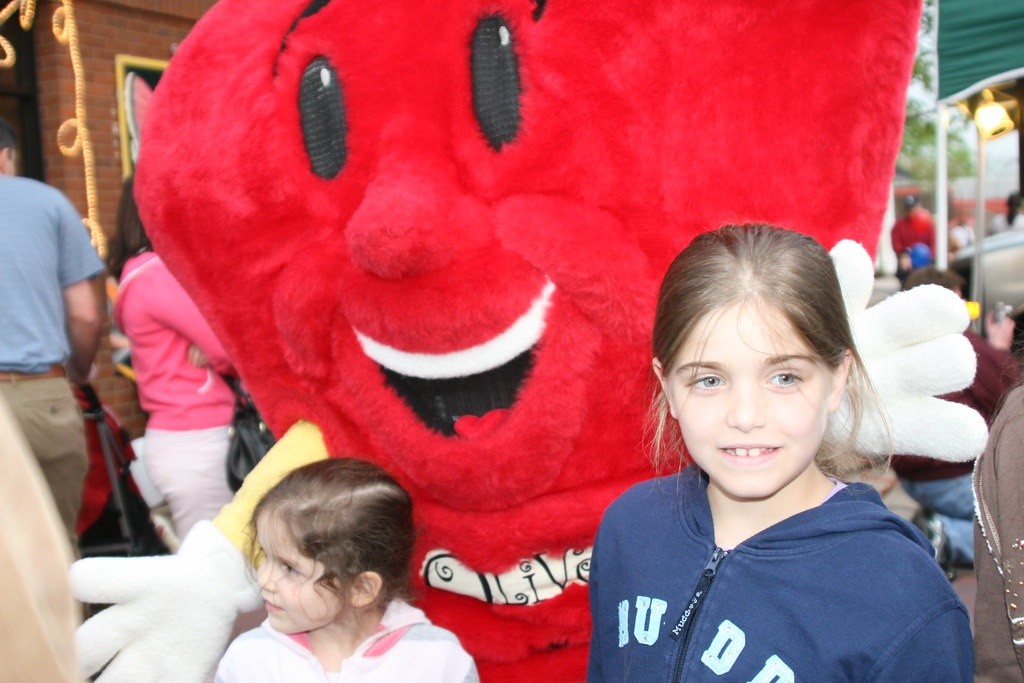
[{"xmin": 74, "ymin": 0, "xmax": 994, "ymax": 683}]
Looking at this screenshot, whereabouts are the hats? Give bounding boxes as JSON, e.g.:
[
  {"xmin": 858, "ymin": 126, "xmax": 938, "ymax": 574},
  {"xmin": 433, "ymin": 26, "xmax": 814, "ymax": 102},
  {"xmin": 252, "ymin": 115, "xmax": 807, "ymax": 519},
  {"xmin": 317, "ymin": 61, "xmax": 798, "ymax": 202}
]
[
  {"xmin": 905, "ymin": 195, "xmax": 917, "ymax": 207},
  {"xmin": 908, "ymin": 243, "xmax": 931, "ymax": 268}
]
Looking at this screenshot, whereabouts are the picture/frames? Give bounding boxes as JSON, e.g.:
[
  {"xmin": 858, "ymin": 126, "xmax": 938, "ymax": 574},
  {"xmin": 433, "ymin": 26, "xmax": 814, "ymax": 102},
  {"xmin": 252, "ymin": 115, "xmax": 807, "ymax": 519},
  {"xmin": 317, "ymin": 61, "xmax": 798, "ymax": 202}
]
[{"xmin": 115, "ymin": 53, "xmax": 168, "ymax": 182}]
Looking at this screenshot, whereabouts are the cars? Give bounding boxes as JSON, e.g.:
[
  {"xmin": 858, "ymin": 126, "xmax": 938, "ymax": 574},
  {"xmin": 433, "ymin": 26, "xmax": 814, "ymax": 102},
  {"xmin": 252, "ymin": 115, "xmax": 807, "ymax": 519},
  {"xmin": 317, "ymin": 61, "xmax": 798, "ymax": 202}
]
[{"xmin": 947, "ymin": 227, "xmax": 1024, "ymax": 357}]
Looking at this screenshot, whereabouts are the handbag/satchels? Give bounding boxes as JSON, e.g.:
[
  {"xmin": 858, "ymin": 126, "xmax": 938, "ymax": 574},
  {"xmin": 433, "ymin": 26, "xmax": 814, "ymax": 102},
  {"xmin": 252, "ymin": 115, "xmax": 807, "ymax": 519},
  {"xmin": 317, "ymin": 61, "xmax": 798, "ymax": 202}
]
[{"xmin": 224, "ymin": 376, "xmax": 276, "ymax": 493}]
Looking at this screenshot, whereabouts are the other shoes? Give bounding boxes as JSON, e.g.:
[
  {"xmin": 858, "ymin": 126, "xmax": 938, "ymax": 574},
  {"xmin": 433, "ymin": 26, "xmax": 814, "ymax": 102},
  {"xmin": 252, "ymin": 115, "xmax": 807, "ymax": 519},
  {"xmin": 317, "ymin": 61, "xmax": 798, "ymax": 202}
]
[{"xmin": 913, "ymin": 511, "xmax": 956, "ymax": 582}]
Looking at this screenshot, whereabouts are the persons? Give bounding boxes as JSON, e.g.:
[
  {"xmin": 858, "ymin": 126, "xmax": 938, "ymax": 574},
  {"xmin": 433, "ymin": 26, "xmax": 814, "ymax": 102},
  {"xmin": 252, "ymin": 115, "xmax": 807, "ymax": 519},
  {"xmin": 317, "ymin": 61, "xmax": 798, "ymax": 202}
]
[
  {"xmin": 0, "ymin": 115, "xmax": 104, "ymax": 569},
  {"xmin": 213, "ymin": 456, "xmax": 480, "ymax": 683},
  {"xmin": 884, "ymin": 191, "xmax": 1024, "ymax": 683},
  {"xmin": 585, "ymin": 224, "xmax": 976, "ymax": 683},
  {"xmin": 107, "ymin": 172, "xmax": 240, "ymax": 556}
]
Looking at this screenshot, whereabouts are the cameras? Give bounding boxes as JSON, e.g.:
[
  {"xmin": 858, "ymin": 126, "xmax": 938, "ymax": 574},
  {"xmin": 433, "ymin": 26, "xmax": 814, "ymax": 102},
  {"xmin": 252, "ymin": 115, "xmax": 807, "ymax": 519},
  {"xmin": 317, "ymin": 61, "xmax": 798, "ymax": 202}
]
[{"xmin": 993, "ymin": 301, "xmax": 1013, "ymax": 321}]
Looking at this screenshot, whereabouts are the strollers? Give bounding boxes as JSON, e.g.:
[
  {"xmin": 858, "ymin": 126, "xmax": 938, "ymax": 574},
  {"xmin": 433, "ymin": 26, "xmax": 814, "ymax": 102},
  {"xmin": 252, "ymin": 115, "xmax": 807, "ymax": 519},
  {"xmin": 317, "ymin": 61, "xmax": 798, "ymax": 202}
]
[{"xmin": 63, "ymin": 377, "xmax": 168, "ymax": 559}]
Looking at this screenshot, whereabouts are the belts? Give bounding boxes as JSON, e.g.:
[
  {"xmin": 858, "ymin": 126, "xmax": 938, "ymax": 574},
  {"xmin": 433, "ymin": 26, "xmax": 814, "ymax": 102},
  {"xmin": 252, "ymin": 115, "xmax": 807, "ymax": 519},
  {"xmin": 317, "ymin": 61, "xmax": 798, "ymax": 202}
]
[{"xmin": 0, "ymin": 364, "xmax": 64, "ymax": 382}]
